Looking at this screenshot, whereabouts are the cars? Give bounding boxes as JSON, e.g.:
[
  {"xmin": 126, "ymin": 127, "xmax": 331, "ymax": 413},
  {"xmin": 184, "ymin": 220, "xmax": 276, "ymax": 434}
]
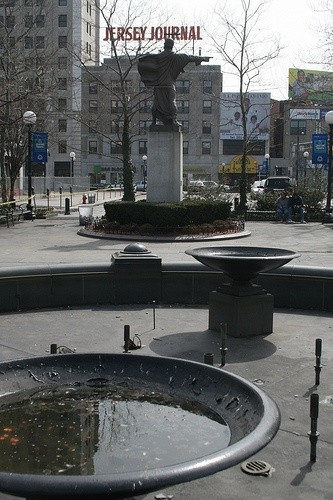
[
  {"xmin": 131, "ymin": 180, "xmax": 147, "ymax": 193},
  {"xmin": 249, "ymin": 181, "xmax": 260, "ymax": 199},
  {"xmin": 257, "ymin": 176, "xmax": 291, "ymax": 196}
]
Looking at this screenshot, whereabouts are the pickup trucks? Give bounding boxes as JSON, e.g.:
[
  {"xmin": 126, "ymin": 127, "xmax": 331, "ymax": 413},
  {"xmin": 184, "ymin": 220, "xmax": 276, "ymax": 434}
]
[{"xmin": 188, "ymin": 180, "xmax": 230, "ymax": 193}]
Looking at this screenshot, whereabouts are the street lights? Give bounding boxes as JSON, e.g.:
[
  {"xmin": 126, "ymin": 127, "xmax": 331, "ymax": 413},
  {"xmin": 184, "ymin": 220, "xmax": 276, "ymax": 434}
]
[
  {"xmin": 221, "ymin": 163, "xmax": 225, "ymax": 174},
  {"xmin": 69, "ymin": 152, "xmax": 76, "ymax": 176},
  {"xmin": 264, "ymin": 153, "xmax": 269, "ymax": 177},
  {"xmin": 325, "ymin": 110, "xmax": 333, "ymax": 212},
  {"xmin": 22, "ymin": 111, "xmax": 38, "ymax": 220},
  {"xmin": 142, "ymin": 155, "xmax": 147, "ymax": 191},
  {"xmin": 296, "ymin": 127, "xmax": 305, "ymax": 148},
  {"xmin": 303, "ymin": 151, "xmax": 309, "ymax": 180}
]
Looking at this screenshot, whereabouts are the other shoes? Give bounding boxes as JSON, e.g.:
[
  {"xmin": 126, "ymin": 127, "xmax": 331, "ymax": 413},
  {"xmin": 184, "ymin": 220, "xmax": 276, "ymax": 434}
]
[
  {"xmin": 288, "ymin": 219, "xmax": 292, "ymax": 223},
  {"xmin": 301, "ymin": 220, "xmax": 306, "ymax": 224}
]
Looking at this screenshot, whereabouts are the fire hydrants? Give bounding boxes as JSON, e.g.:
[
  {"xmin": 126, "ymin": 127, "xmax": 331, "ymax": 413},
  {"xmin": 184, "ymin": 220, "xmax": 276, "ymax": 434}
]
[{"xmin": 82, "ymin": 193, "xmax": 87, "ymax": 204}]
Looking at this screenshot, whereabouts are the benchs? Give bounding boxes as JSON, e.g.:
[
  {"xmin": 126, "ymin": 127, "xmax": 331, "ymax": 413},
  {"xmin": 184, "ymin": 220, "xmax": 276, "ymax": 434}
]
[
  {"xmin": 0, "ymin": 203, "xmax": 32, "ymax": 228},
  {"xmin": 246, "ymin": 205, "xmax": 307, "ymax": 222}
]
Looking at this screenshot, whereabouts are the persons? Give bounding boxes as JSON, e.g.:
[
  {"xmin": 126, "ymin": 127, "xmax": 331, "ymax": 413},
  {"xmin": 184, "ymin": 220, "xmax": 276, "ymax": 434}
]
[
  {"xmin": 289, "ymin": 70, "xmax": 309, "ymax": 99},
  {"xmin": 230, "ymin": 97, "xmax": 259, "ymax": 134},
  {"xmin": 138, "ymin": 38, "xmax": 214, "ymax": 127},
  {"xmin": 277, "ymin": 190, "xmax": 306, "ymax": 224}
]
